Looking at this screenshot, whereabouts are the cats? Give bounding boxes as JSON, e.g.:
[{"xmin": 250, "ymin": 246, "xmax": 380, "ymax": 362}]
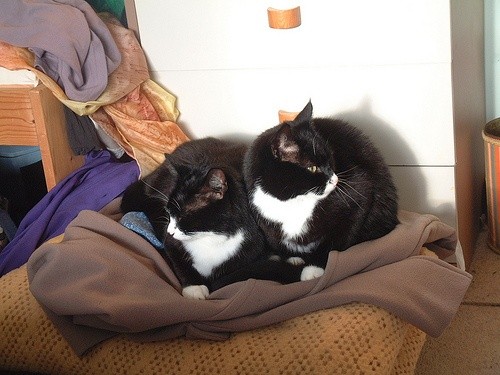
[{"xmin": 120, "ymin": 99, "xmax": 401, "ymax": 302}]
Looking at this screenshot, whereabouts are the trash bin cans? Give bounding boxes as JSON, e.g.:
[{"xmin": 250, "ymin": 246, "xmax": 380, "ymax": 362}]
[{"xmin": 482, "ymin": 117, "xmax": 500, "ymax": 255}]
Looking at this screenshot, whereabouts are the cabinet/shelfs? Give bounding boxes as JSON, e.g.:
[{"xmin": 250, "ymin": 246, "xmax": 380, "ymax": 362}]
[{"xmin": 124, "ymin": 0, "xmax": 485, "ymax": 271}]
